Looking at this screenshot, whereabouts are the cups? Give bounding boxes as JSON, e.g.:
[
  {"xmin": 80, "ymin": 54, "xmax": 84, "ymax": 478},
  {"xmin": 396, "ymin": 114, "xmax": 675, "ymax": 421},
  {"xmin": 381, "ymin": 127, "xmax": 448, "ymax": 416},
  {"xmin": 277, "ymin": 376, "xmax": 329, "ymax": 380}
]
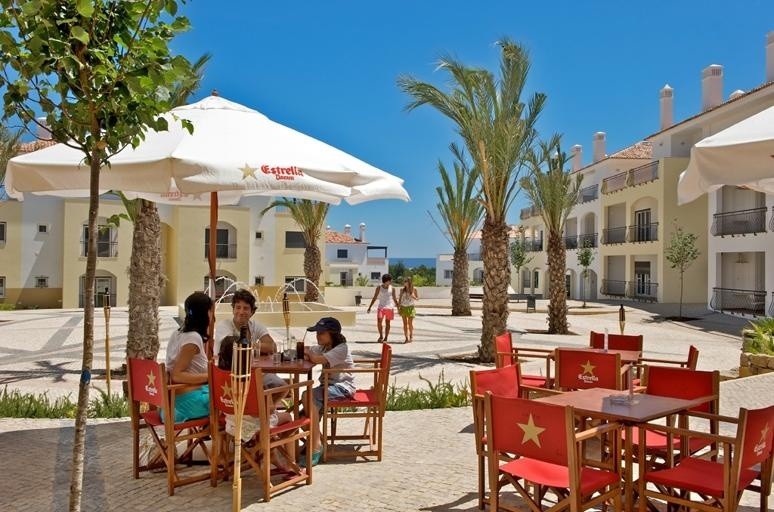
[
  {"xmin": 282, "ymin": 338, "xmax": 291, "ymax": 361},
  {"xmin": 250, "ymin": 340, "xmax": 261, "ymax": 363},
  {"xmin": 272, "ymin": 341, "xmax": 281, "ymax": 363}
]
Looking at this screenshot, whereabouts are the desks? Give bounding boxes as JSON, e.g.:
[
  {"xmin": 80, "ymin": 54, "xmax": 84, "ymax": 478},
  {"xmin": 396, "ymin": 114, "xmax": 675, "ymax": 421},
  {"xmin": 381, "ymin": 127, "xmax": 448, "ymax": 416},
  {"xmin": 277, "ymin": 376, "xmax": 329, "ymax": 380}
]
[
  {"xmin": 251, "ymin": 360, "xmax": 317, "ymax": 463},
  {"xmin": 532, "ymin": 387, "xmax": 700, "ymax": 512}
]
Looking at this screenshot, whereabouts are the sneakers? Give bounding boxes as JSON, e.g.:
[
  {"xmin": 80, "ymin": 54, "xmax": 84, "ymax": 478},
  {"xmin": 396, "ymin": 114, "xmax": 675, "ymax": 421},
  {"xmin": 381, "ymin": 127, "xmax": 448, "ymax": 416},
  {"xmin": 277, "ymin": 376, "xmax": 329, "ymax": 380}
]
[{"xmin": 300, "ymin": 445, "xmax": 323, "ymax": 467}]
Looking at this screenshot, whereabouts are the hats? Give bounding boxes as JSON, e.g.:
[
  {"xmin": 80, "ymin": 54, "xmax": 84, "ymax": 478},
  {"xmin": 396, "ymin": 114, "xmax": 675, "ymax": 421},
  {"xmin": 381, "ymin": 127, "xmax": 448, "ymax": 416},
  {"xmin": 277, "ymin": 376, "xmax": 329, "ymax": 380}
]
[{"xmin": 307, "ymin": 318, "xmax": 340, "ymax": 332}]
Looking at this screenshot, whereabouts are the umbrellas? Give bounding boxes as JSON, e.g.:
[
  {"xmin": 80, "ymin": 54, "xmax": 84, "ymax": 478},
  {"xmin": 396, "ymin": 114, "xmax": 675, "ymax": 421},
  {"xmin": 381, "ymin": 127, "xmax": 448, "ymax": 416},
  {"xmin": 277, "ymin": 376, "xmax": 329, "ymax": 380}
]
[{"xmin": 2, "ymin": 89, "xmax": 410, "ymax": 356}]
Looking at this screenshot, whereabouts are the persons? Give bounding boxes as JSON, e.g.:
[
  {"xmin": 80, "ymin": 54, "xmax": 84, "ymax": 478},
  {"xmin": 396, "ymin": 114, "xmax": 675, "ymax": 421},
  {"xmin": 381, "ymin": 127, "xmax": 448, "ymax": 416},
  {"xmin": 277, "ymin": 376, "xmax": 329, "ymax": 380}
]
[
  {"xmin": 215, "ymin": 335, "xmax": 309, "ymax": 481},
  {"xmin": 366, "ymin": 274, "xmax": 400, "ymax": 343},
  {"xmin": 216, "ymin": 290, "xmax": 288, "ymax": 406},
  {"xmin": 399, "ymin": 279, "xmax": 419, "ymax": 342},
  {"xmin": 298, "ymin": 317, "xmax": 357, "ymax": 467},
  {"xmin": 159, "ymin": 292, "xmax": 213, "ymax": 425}
]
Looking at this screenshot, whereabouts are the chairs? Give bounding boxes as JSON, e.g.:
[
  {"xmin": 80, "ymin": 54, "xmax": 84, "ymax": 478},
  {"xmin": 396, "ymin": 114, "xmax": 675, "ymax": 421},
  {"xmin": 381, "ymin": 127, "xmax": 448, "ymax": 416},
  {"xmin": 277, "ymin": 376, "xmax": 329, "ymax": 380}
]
[
  {"xmin": 548, "ymin": 349, "xmax": 641, "ymax": 390},
  {"xmin": 126, "ymin": 355, "xmax": 230, "ymax": 497},
  {"xmin": 593, "ymin": 333, "xmax": 605, "ymax": 350},
  {"xmin": 590, "ymin": 330, "xmax": 596, "ymax": 348},
  {"xmin": 634, "ymin": 404, "xmax": 774, "ymax": 512},
  {"xmin": 608, "ymin": 335, "xmax": 643, "ymax": 386},
  {"xmin": 207, "ymin": 361, "xmax": 314, "ymax": 503},
  {"xmin": 322, "ymin": 340, "xmax": 392, "ymax": 463},
  {"xmin": 600, "ymin": 364, "xmax": 720, "ymax": 512},
  {"xmin": 555, "ymin": 347, "xmax": 633, "ymax": 391},
  {"xmin": 469, "ymin": 362, "xmax": 568, "ymax": 511},
  {"xmin": 636, "ymin": 344, "xmax": 699, "ymax": 372},
  {"xmin": 493, "ymin": 332, "xmax": 553, "ymax": 400},
  {"xmin": 484, "ymin": 390, "xmax": 625, "ymax": 511}
]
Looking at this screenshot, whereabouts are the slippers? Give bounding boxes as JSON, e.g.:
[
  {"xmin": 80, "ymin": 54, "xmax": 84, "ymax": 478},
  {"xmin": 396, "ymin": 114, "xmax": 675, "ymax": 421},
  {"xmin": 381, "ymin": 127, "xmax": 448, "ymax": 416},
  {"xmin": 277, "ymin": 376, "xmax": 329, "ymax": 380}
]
[
  {"xmin": 270, "ymin": 465, "xmax": 294, "ymax": 476},
  {"xmin": 283, "ymin": 468, "xmax": 306, "ymax": 481}
]
